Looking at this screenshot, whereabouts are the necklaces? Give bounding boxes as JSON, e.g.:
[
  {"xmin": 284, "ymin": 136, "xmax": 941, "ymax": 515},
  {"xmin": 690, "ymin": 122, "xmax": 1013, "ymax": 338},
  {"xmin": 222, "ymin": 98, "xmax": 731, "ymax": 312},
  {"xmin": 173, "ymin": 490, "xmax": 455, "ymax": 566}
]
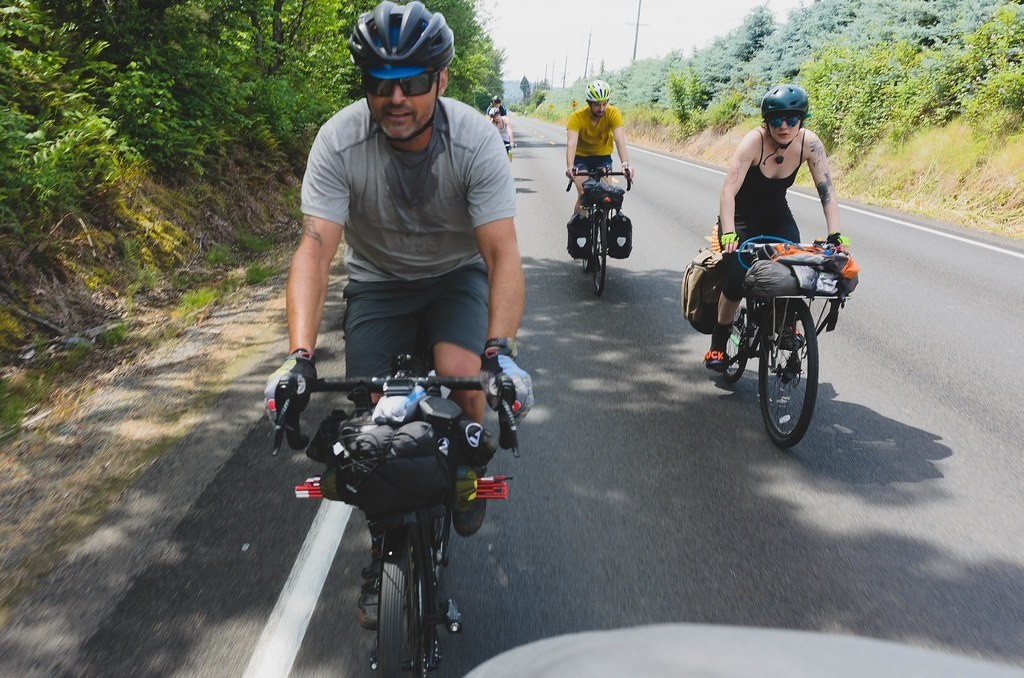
[{"xmin": 769, "ymin": 132, "xmax": 794, "ymax": 164}]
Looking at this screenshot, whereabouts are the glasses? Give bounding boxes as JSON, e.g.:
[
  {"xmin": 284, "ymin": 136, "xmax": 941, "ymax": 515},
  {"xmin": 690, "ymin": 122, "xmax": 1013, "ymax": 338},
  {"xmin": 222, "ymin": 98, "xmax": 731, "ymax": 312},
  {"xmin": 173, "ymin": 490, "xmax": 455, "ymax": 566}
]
[
  {"xmin": 766, "ymin": 116, "xmax": 800, "ymax": 128},
  {"xmin": 361, "ymin": 71, "xmax": 440, "ymax": 97}
]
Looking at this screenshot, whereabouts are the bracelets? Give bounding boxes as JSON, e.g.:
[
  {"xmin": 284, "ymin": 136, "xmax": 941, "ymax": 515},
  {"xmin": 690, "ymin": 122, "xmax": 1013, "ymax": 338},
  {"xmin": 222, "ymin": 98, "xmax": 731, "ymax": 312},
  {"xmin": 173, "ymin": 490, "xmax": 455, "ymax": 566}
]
[{"xmin": 622, "ymin": 161, "xmax": 630, "ymax": 167}]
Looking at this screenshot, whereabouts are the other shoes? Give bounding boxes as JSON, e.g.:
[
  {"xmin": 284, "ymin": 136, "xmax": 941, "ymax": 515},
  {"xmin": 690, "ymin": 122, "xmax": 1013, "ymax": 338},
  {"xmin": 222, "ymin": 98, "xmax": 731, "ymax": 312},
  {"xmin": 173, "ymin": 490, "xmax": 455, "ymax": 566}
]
[
  {"xmin": 706, "ymin": 350, "xmax": 730, "ymax": 369},
  {"xmin": 784, "ymin": 328, "xmax": 804, "ymax": 348}
]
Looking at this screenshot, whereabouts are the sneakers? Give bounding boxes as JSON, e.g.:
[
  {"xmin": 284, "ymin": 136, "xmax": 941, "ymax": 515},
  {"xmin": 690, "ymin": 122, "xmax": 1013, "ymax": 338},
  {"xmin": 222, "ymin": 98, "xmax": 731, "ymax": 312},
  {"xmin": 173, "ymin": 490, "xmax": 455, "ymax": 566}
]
[
  {"xmin": 356, "ymin": 558, "xmax": 382, "ymax": 628},
  {"xmin": 454, "ymin": 465, "xmax": 488, "ymax": 536},
  {"xmin": 447, "ymin": 416, "xmax": 498, "ymax": 467}
]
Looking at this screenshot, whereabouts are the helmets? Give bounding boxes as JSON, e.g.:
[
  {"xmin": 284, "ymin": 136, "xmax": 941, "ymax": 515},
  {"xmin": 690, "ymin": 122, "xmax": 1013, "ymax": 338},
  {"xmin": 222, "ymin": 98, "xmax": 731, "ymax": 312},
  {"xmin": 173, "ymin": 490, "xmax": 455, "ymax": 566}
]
[
  {"xmin": 347, "ymin": 1, "xmax": 454, "ymax": 79},
  {"xmin": 491, "ymin": 96, "xmax": 501, "ymax": 104},
  {"xmin": 761, "ymin": 84, "xmax": 809, "ymax": 120},
  {"xmin": 489, "ymin": 107, "xmax": 500, "ymax": 117},
  {"xmin": 586, "ymin": 81, "xmax": 612, "ymax": 101}
]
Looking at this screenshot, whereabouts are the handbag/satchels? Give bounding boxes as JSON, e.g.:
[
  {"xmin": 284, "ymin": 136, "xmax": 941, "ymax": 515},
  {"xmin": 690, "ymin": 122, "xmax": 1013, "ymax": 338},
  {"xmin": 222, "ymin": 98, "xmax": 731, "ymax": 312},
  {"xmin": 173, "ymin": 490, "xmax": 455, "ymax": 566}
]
[
  {"xmin": 679, "ymin": 249, "xmax": 721, "ymax": 334},
  {"xmin": 335, "ymin": 450, "xmax": 452, "ymax": 513},
  {"xmin": 578, "ymin": 178, "xmax": 624, "ymax": 209},
  {"xmin": 772, "ymin": 230, "xmax": 859, "ymax": 333}
]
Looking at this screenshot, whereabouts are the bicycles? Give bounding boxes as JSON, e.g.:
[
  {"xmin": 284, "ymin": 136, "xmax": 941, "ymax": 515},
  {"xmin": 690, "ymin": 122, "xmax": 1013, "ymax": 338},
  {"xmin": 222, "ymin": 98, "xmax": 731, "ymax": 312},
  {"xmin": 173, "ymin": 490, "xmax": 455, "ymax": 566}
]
[
  {"xmin": 268, "ymin": 345, "xmax": 523, "ymax": 678},
  {"xmin": 565, "ymin": 167, "xmax": 633, "ymax": 298},
  {"xmin": 712, "ymin": 222, "xmax": 844, "ymax": 449}
]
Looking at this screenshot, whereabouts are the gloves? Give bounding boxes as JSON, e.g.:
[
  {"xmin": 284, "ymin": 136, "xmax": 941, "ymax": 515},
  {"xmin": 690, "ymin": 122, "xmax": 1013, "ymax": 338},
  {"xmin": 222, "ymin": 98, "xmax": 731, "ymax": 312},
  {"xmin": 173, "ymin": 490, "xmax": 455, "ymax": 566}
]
[
  {"xmin": 265, "ymin": 352, "xmax": 316, "ymax": 431},
  {"xmin": 477, "ymin": 352, "xmax": 536, "ymax": 427}
]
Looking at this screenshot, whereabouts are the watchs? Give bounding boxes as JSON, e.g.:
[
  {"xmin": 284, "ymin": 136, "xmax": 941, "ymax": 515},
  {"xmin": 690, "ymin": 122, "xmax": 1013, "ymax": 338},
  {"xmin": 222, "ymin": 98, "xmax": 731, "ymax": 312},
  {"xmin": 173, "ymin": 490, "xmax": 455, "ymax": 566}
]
[{"xmin": 484, "ymin": 336, "xmax": 518, "ymax": 361}]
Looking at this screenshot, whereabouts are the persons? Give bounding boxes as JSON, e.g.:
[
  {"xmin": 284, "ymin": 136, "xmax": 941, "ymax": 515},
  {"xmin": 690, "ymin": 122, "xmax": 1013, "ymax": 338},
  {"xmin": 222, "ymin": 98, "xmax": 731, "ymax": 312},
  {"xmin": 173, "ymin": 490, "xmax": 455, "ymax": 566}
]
[
  {"xmin": 260, "ymin": 1, "xmax": 536, "ymax": 631},
  {"xmin": 704, "ymin": 85, "xmax": 851, "ymax": 372},
  {"xmin": 566, "ymin": 80, "xmax": 634, "ymax": 218},
  {"xmin": 487, "ymin": 94, "xmax": 513, "ymax": 159}
]
